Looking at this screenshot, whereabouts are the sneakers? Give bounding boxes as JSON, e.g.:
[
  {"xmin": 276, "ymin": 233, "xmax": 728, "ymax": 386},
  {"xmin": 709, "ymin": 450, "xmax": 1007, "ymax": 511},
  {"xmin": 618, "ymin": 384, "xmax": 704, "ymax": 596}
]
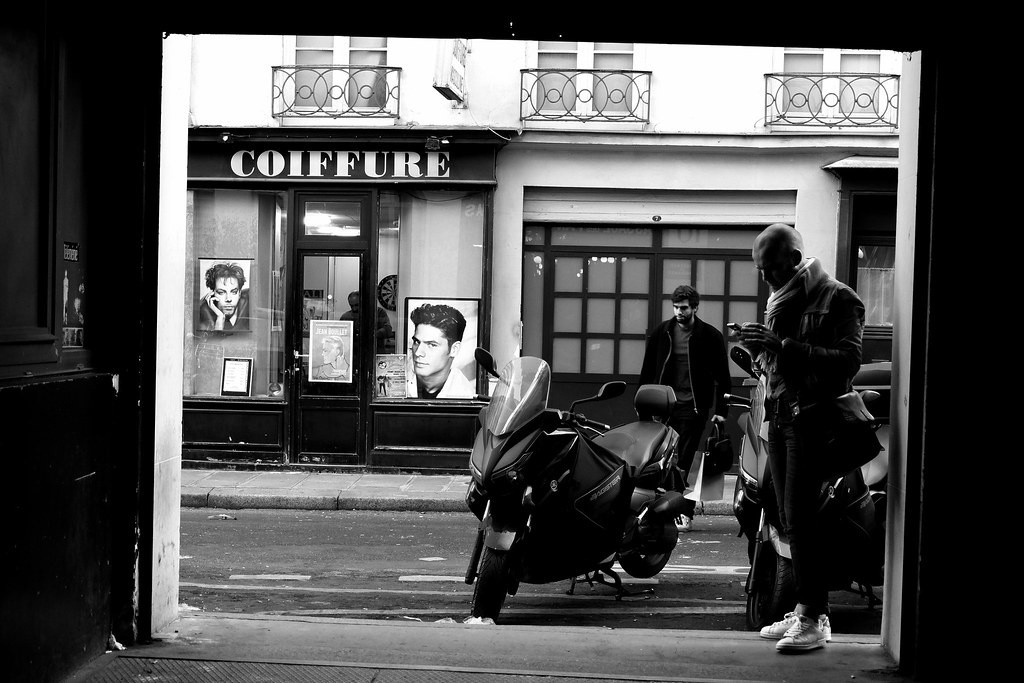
[
  {"xmin": 674, "ymin": 514, "xmax": 692, "ymax": 531},
  {"xmin": 775, "ymin": 614, "xmax": 826, "ymax": 650},
  {"xmin": 760, "ymin": 603, "xmax": 826, "ymax": 638}
]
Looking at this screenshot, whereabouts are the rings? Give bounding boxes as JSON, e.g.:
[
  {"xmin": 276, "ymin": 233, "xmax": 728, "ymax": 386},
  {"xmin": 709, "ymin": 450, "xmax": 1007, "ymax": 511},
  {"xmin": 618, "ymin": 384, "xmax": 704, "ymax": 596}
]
[{"xmin": 759, "ymin": 329, "xmax": 762, "ymax": 333}]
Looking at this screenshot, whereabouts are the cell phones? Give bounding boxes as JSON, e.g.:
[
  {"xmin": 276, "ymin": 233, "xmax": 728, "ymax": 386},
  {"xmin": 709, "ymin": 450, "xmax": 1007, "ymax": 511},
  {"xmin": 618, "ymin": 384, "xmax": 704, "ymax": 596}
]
[{"xmin": 727, "ymin": 322, "xmax": 743, "ymax": 332}]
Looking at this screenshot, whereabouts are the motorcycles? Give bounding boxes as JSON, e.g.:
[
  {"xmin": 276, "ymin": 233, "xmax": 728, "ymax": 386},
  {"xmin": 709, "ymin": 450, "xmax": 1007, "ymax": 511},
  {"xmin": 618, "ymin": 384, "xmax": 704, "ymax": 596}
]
[
  {"xmin": 462, "ymin": 345, "xmax": 698, "ymax": 623},
  {"xmin": 723, "ymin": 344, "xmax": 889, "ymax": 641}
]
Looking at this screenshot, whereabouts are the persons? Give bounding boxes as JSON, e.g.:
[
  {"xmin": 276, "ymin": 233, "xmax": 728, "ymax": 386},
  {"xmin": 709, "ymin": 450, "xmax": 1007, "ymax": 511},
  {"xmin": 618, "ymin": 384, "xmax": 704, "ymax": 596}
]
[
  {"xmin": 410, "ymin": 305, "xmax": 474, "ymax": 399},
  {"xmin": 638, "ymin": 285, "xmax": 732, "ymax": 531},
  {"xmin": 340, "ymin": 290, "xmax": 392, "ymax": 369},
  {"xmin": 198, "ymin": 264, "xmax": 249, "ymax": 330},
  {"xmin": 732, "ymin": 223, "xmax": 866, "ymax": 650}
]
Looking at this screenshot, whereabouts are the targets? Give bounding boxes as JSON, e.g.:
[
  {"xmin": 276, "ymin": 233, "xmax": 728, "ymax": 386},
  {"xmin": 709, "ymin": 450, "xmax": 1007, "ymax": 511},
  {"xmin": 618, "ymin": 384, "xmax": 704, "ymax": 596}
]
[{"xmin": 376, "ymin": 274, "xmax": 398, "ymax": 312}]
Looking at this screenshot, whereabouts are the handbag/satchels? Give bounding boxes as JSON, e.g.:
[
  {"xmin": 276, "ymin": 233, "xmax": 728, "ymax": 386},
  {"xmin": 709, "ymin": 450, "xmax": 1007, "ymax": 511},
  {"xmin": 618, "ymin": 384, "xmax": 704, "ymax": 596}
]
[
  {"xmin": 682, "ymin": 422, "xmax": 725, "ymax": 501},
  {"xmin": 704, "ymin": 419, "xmax": 733, "ymax": 477},
  {"xmin": 790, "ymin": 389, "xmax": 880, "ymax": 478}
]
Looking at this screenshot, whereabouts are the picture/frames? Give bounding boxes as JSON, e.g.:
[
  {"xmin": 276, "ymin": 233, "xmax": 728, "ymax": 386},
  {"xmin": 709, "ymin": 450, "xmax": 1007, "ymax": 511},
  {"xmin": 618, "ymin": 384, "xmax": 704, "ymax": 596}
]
[
  {"xmin": 376, "ymin": 354, "xmax": 407, "ymax": 399},
  {"xmin": 219, "ymin": 357, "xmax": 253, "ymax": 397},
  {"xmin": 404, "ymin": 297, "xmax": 480, "ymax": 400}
]
